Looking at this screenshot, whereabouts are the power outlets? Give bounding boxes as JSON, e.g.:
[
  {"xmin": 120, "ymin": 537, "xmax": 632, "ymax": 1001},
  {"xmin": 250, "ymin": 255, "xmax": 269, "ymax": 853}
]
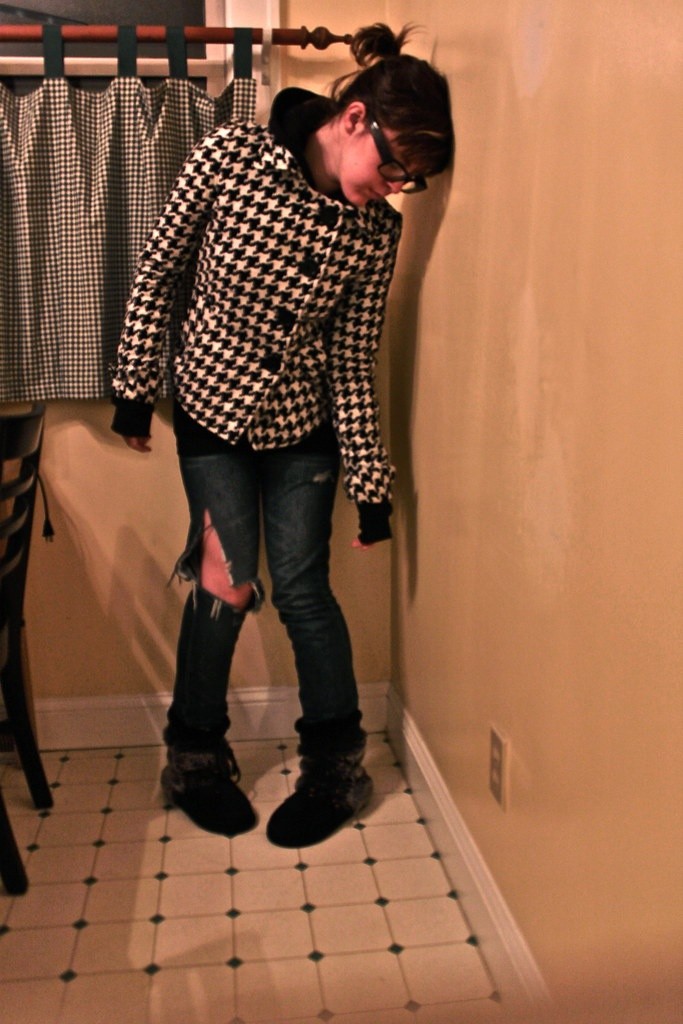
[{"xmin": 489, "ymin": 725, "xmax": 505, "ymax": 807}]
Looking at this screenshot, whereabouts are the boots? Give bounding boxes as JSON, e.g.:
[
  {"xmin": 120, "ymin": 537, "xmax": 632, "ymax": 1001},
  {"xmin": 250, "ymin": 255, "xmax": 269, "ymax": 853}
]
[
  {"xmin": 160, "ymin": 714, "xmax": 259, "ymax": 838},
  {"xmin": 266, "ymin": 710, "xmax": 373, "ymax": 849}
]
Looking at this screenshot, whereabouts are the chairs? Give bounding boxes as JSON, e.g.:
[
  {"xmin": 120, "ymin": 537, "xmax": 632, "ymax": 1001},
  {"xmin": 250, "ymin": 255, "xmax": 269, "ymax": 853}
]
[{"xmin": 0, "ymin": 398, "xmax": 53, "ymax": 894}]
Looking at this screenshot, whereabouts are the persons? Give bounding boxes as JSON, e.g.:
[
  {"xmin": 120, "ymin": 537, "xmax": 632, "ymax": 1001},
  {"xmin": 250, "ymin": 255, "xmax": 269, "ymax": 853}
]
[{"xmin": 108, "ymin": 22, "xmax": 457, "ymax": 848}]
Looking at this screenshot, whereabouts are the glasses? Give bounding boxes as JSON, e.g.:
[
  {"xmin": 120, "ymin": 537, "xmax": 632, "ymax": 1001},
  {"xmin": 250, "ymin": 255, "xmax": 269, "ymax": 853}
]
[{"xmin": 364, "ymin": 112, "xmax": 429, "ymax": 195}]
